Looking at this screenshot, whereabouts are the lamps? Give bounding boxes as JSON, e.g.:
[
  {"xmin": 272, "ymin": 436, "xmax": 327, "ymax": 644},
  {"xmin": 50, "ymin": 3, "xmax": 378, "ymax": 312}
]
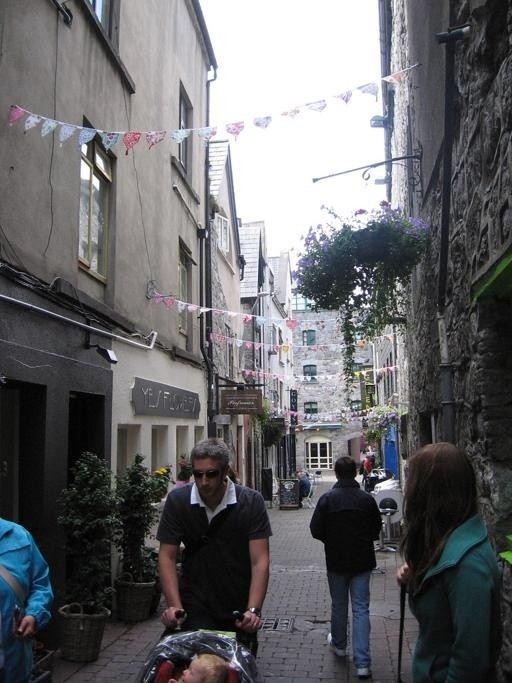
[{"xmin": 83, "ymin": 342, "xmax": 119, "ymax": 365}]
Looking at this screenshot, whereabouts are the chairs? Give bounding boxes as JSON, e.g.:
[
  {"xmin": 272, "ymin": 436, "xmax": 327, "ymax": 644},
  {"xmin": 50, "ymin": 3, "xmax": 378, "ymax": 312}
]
[{"xmin": 301, "ymin": 484, "xmax": 318, "ymax": 510}]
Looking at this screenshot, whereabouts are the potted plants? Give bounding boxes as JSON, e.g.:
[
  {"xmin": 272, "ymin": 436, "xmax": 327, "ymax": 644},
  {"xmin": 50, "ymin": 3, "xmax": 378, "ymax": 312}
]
[{"xmin": 50, "ymin": 449, "xmax": 196, "ymax": 664}]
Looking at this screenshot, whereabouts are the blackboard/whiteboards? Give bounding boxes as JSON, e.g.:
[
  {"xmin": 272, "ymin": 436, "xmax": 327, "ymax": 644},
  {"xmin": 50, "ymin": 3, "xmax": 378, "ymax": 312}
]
[{"xmin": 279, "ymin": 479, "xmax": 300, "ymax": 508}]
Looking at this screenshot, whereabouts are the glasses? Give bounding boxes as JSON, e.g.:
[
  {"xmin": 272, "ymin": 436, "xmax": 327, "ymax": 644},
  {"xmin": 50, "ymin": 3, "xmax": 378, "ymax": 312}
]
[{"xmin": 192, "ymin": 469, "xmax": 224, "ymax": 479}]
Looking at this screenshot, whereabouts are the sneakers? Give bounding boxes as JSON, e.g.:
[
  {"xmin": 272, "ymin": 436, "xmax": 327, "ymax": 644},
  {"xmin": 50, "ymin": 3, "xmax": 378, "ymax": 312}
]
[
  {"xmin": 357, "ymin": 667, "xmax": 373, "ymax": 677},
  {"xmin": 326, "ymin": 633, "xmax": 347, "ymax": 657}
]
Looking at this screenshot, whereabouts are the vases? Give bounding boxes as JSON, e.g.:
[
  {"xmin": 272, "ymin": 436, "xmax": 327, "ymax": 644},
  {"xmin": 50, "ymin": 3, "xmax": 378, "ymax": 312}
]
[{"xmin": 348, "ymin": 221, "xmax": 389, "ymax": 266}]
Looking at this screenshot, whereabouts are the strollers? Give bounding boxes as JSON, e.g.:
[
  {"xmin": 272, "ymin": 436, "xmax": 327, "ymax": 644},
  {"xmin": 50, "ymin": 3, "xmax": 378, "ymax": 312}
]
[{"xmin": 134, "ymin": 608, "xmax": 261, "ymax": 683}]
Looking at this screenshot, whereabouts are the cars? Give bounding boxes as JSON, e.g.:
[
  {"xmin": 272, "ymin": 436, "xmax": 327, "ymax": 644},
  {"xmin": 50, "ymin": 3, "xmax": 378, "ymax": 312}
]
[{"xmin": 364, "ymin": 467, "xmax": 403, "ymax": 514}]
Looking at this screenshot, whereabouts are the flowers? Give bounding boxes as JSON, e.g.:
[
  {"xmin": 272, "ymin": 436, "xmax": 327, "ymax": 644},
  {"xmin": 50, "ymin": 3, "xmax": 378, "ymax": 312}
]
[{"xmin": 285, "ymin": 197, "xmax": 436, "ymax": 410}]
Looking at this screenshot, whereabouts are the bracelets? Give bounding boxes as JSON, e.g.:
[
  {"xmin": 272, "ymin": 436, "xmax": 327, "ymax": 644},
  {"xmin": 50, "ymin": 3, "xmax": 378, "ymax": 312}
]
[{"xmin": 244, "ymin": 607, "xmax": 261, "ymax": 617}]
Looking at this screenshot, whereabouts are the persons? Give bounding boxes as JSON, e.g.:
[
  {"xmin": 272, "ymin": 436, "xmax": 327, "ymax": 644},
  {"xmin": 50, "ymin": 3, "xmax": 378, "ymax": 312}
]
[
  {"xmin": 296, "ymin": 470, "xmax": 311, "ymax": 507},
  {"xmin": 156, "ymin": 437, "xmax": 273, "ymax": 661},
  {"xmin": 392, "ymin": 442, "xmax": 504, "ymax": 682},
  {"xmin": 309, "ymin": 456, "xmax": 382, "ymax": 679},
  {"xmin": 166, "ymin": 653, "xmax": 229, "ymax": 683},
  {"xmin": 0, "ymin": 518, "xmax": 55, "ymax": 682},
  {"xmin": 360, "ymin": 454, "xmax": 373, "ymax": 485}
]
